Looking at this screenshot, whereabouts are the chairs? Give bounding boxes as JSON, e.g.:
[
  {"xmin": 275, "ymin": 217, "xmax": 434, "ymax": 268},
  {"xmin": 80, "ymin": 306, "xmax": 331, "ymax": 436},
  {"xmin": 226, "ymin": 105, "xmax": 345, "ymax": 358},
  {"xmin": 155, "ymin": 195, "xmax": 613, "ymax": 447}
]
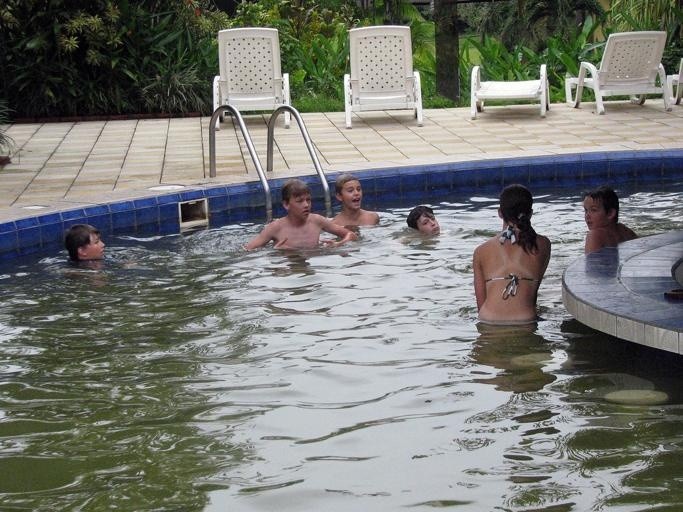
[
  {"xmin": 343, "ymin": 26, "xmax": 424, "ymax": 129},
  {"xmin": 565, "ymin": 31, "xmax": 673, "ymax": 114},
  {"xmin": 212, "ymin": 27, "xmax": 291, "ymax": 130},
  {"xmin": 471, "ymin": 64, "xmax": 551, "ymax": 121},
  {"xmin": 667, "ymin": 57, "xmax": 683, "ymax": 105}
]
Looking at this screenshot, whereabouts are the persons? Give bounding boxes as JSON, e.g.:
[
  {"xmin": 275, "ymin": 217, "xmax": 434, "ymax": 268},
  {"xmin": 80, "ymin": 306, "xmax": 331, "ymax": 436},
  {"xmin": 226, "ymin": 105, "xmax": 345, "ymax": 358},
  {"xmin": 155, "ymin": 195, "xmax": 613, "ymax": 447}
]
[
  {"xmin": 241, "ymin": 178, "xmax": 358, "ymax": 250},
  {"xmin": 406, "ymin": 206, "xmax": 441, "ymax": 236},
  {"xmin": 582, "ymin": 185, "xmax": 639, "ymax": 255},
  {"xmin": 64, "ymin": 223, "xmax": 105, "ymax": 260},
  {"xmin": 471, "ymin": 183, "xmax": 550, "ymax": 324},
  {"xmin": 328, "ymin": 174, "xmax": 379, "ymax": 227}
]
